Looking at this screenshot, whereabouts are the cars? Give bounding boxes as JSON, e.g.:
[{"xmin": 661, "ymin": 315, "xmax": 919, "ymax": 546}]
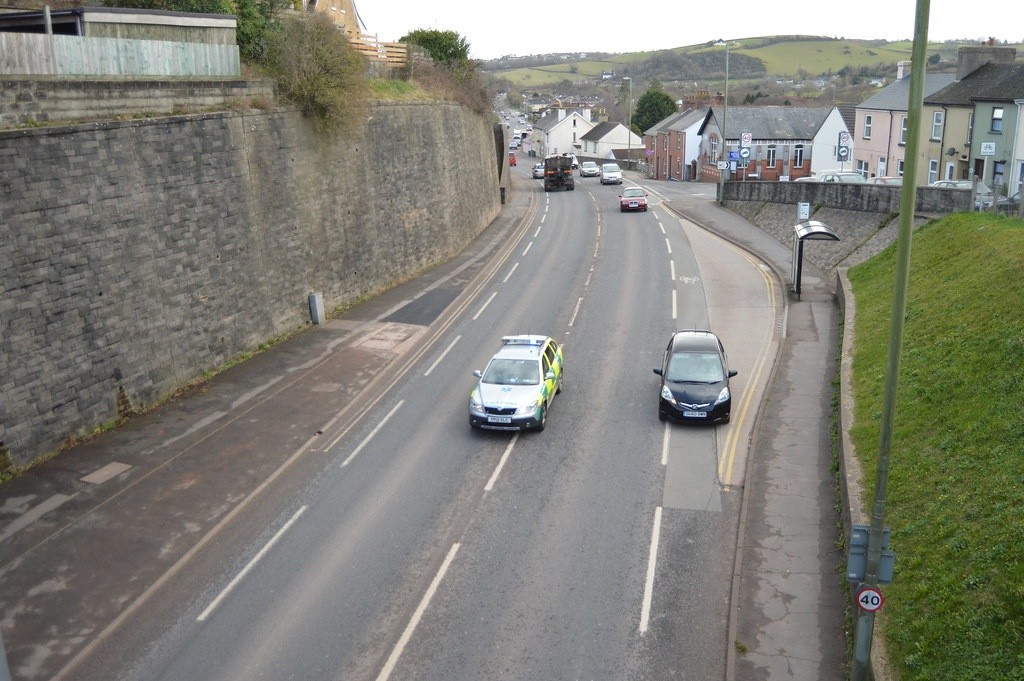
[
  {"xmin": 793, "ymin": 172, "xmax": 1024, "ymax": 218},
  {"xmin": 511, "ymin": 125, "xmax": 533, "ymax": 146},
  {"xmin": 508, "ymin": 153, "xmax": 516, "ymax": 165},
  {"xmin": 579, "ymin": 162, "xmax": 600, "ymax": 176},
  {"xmin": 532, "ymin": 163, "xmax": 545, "ymax": 178},
  {"xmin": 563, "ymin": 154, "xmax": 577, "ymax": 168},
  {"xmin": 509, "ymin": 140, "xmax": 518, "ymax": 149},
  {"xmin": 618, "ymin": 187, "xmax": 648, "ymax": 212},
  {"xmin": 518, "ymin": 117, "xmax": 526, "ymax": 124},
  {"xmin": 469, "ymin": 335, "xmax": 563, "ymax": 431},
  {"xmin": 491, "ymin": 93, "xmax": 515, "ymax": 120},
  {"xmin": 653, "ymin": 330, "xmax": 738, "ymax": 425}
]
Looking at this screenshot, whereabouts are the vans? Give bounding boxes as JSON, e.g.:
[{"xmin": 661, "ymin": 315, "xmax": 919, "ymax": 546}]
[{"xmin": 600, "ymin": 162, "xmax": 623, "ymax": 184}]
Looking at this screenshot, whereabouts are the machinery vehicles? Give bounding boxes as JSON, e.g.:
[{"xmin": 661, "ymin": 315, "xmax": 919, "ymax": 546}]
[{"xmin": 541, "ymin": 156, "xmax": 574, "ymax": 191}]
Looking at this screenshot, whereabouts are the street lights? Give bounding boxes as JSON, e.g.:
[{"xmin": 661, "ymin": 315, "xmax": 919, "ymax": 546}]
[
  {"xmin": 714, "ymin": 42, "xmax": 729, "ymax": 205},
  {"xmin": 623, "ymin": 77, "xmax": 632, "ymax": 169}
]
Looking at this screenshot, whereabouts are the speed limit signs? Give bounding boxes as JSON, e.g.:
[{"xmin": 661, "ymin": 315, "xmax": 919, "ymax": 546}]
[
  {"xmin": 742, "ymin": 134, "xmax": 751, "ymax": 147},
  {"xmin": 839, "ymin": 131, "xmax": 849, "ymax": 146},
  {"xmin": 856, "ymin": 588, "xmax": 883, "ymax": 612}
]
